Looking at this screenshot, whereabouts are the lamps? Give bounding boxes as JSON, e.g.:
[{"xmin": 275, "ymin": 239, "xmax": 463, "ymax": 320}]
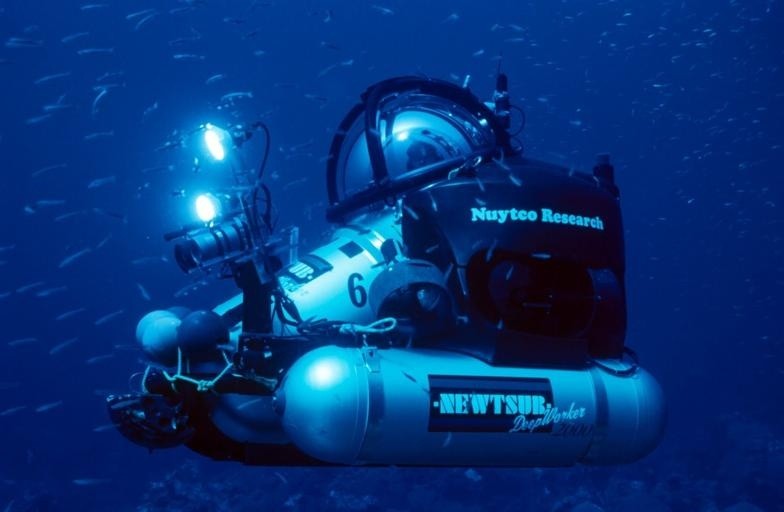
[{"xmin": 188, "ymin": 109, "xmax": 256, "ymax": 225}]
